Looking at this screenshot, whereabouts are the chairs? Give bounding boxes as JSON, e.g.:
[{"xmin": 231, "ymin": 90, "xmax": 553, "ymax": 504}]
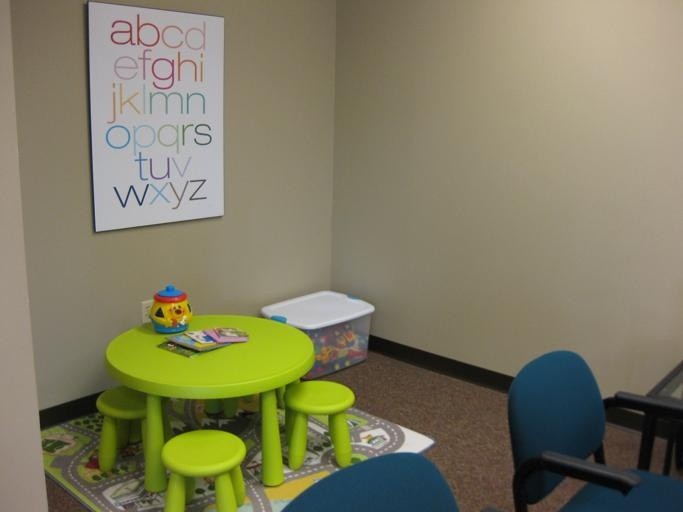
[
  {"xmin": 282, "ymin": 451, "xmax": 460, "ymax": 511},
  {"xmin": 506, "ymin": 350, "xmax": 682, "ymax": 512}
]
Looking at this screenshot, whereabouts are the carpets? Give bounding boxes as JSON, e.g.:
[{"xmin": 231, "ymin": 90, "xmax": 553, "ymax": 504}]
[{"xmin": 39, "ymin": 393, "xmax": 436, "ymax": 512}]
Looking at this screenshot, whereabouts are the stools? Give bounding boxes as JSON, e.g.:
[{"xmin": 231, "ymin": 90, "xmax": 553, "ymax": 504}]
[{"xmin": 93, "ymin": 381, "xmax": 355, "ymax": 512}]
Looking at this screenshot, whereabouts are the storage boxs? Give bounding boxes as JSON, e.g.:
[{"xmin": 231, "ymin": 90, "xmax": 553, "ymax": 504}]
[{"xmin": 260, "ymin": 289, "xmax": 375, "ymax": 382}]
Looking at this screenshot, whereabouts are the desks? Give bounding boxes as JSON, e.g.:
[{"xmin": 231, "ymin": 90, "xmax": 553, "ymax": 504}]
[{"xmin": 106, "ymin": 313, "xmax": 315, "ymax": 494}]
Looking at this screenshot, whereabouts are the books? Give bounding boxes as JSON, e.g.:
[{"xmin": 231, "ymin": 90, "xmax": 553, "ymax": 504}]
[{"xmin": 157, "ymin": 326, "xmax": 249, "ymax": 358}]
